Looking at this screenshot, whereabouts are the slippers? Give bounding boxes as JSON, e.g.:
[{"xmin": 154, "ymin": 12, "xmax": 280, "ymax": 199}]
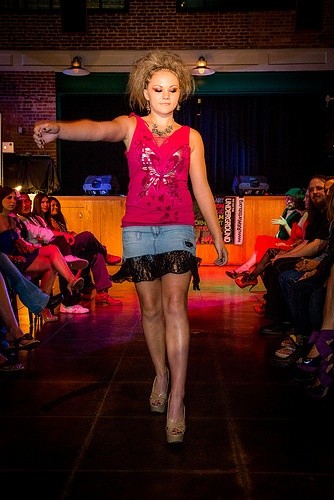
[
  {"xmin": 6, "ymin": 333, "xmax": 40, "ymax": 350},
  {"xmin": 0, "ymin": 360, "xmax": 24, "ymax": 372}
]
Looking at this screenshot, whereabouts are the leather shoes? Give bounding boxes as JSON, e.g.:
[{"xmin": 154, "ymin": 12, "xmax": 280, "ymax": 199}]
[
  {"xmin": 95, "ymin": 294, "xmax": 122, "ymax": 305},
  {"xmin": 104, "ymin": 253, "xmax": 121, "ymax": 265},
  {"xmin": 47, "ymin": 293, "xmax": 65, "ymax": 308}
]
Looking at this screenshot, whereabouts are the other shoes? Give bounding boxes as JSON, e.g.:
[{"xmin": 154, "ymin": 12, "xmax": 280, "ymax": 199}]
[{"xmin": 261, "ymin": 322, "xmax": 306, "ymax": 366}]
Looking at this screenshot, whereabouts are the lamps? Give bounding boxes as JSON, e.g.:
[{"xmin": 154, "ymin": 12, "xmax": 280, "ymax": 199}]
[
  {"xmin": 188, "ymin": 55, "xmax": 216, "ymax": 77},
  {"xmin": 62, "ymin": 55, "xmax": 90, "ymax": 77}
]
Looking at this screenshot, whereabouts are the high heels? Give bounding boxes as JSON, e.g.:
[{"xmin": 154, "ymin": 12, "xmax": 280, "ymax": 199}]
[
  {"xmin": 226, "ymin": 271, "xmax": 250, "ymax": 279},
  {"xmin": 235, "ymin": 276, "xmax": 258, "ymax": 291},
  {"xmin": 165, "ymin": 393, "xmax": 185, "ymax": 443},
  {"xmin": 296, "ymin": 330, "xmax": 334, "ymax": 398},
  {"xmin": 150, "ymin": 367, "xmax": 170, "ymax": 412},
  {"xmin": 254, "ymin": 306, "xmax": 265, "ymax": 314},
  {"xmin": 39, "ymin": 308, "xmax": 58, "ymax": 321},
  {"xmin": 67, "ymin": 278, "xmax": 84, "ymax": 295}
]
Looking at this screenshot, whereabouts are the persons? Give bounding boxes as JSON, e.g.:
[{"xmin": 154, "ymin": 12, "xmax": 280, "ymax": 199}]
[
  {"xmin": 33, "ymin": 52, "xmax": 230, "ymax": 446},
  {"xmin": 226, "ymin": 178, "xmax": 333, "ymax": 401},
  {"xmin": 2, "ymin": 187, "xmax": 124, "ymax": 374}
]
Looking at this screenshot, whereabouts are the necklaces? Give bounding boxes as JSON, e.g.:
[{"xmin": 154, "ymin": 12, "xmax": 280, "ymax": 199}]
[{"xmin": 150, "ymin": 119, "xmax": 177, "ymax": 137}]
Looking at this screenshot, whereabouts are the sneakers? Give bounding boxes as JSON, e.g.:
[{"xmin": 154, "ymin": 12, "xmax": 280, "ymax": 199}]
[
  {"xmin": 64, "ymin": 255, "xmax": 88, "ymax": 269},
  {"xmin": 60, "ymin": 303, "xmax": 89, "ymax": 313}
]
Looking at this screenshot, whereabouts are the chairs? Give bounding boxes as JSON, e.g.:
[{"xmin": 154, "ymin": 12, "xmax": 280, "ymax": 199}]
[{"xmin": 10, "ymin": 263, "xmax": 97, "ymax": 335}]
